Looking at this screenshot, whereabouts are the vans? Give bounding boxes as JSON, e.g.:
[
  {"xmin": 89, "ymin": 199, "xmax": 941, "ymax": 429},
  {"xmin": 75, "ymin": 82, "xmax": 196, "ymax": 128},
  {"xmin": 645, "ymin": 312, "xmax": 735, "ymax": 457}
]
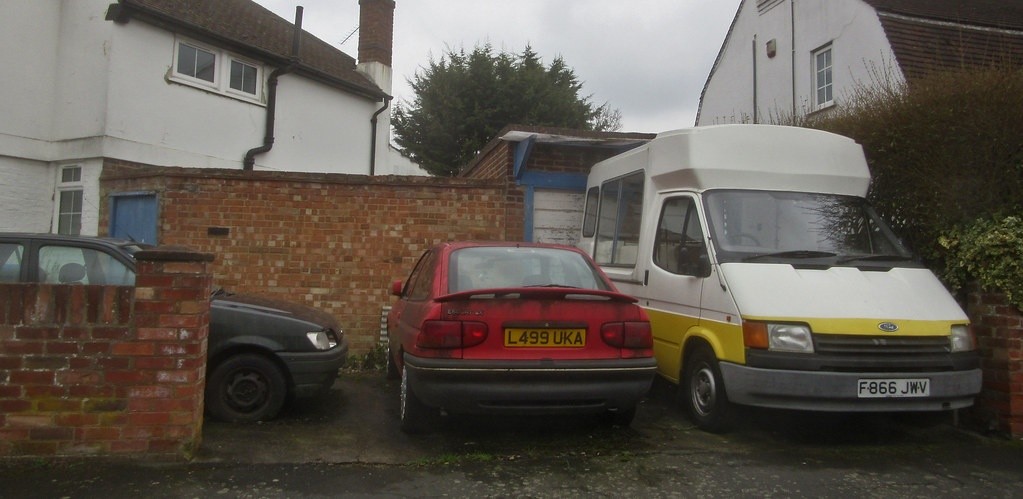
[{"xmin": 576, "ymin": 123, "xmax": 982, "ymax": 432}]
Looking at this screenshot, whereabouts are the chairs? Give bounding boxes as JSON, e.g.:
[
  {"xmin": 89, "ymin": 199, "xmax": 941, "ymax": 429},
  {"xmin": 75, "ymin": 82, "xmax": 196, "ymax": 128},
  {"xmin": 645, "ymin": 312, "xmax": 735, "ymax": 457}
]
[
  {"xmin": 523, "ymin": 275, "xmax": 553, "ymax": 286},
  {"xmin": 59, "ymin": 263, "xmax": 86, "ymax": 285}
]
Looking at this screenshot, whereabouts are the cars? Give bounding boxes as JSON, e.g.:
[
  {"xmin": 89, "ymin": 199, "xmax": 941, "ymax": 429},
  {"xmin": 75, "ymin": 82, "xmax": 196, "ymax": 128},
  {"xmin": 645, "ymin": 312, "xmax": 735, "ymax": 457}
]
[
  {"xmin": 383, "ymin": 241, "xmax": 658, "ymax": 433},
  {"xmin": 0, "ymin": 233, "xmax": 349, "ymax": 429}
]
